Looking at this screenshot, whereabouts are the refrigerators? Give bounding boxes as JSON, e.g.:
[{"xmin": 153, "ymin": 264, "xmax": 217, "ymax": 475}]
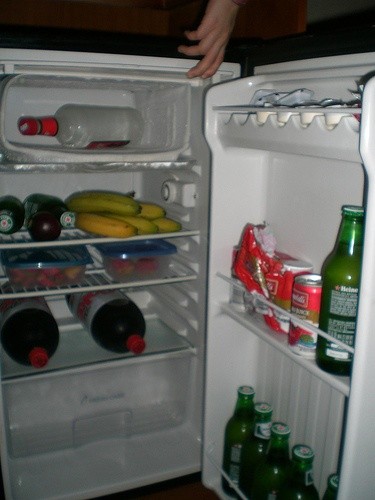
[{"xmin": 0, "ymin": 47, "xmax": 375, "ymax": 500}]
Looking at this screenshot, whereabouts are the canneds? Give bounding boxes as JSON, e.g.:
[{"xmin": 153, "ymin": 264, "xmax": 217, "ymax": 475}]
[{"xmin": 230, "ymin": 246, "xmax": 323, "ymax": 360}]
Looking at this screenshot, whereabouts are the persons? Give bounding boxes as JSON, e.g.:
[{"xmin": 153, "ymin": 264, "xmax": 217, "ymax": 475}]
[{"xmin": 0, "ymin": 0, "xmax": 308, "ymax": 79}]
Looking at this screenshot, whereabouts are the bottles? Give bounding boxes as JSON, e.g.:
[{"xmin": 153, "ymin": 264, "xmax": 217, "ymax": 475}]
[
  {"xmin": 276, "ymin": 444, "xmax": 320, "ymax": 500},
  {"xmin": 0, "ymin": 195, "xmax": 24, "ymax": 235},
  {"xmin": 238, "ymin": 401, "xmax": 273, "ymax": 497},
  {"xmin": 222, "ymin": 384, "xmax": 256, "ymax": 497},
  {"xmin": 323, "ymin": 473, "xmax": 339, "ymax": 500},
  {"xmin": 65, "ymin": 272, "xmax": 146, "ymax": 355},
  {"xmin": 0, "ymin": 281, "xmax": 60, "ymax": 368},
  {"xmin": 252, "ymin": 421, "xmax": 291, "ymax": 499},
  {"xmin": 17, "ymin": 103, "xmax": 144, "ymax": 149},
  {"xmin": 24, "ymin": 193, "xmax": 78, "ymax": 229},
  {"xmin": 317, "ymin": 205, "xmax": 365, "ymax": 376}
]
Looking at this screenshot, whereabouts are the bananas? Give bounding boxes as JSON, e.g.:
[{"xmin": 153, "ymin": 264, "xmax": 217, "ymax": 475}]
[{"xmin": 62, "ymin": 190, "xmax": 181, "ymax": 239}]
